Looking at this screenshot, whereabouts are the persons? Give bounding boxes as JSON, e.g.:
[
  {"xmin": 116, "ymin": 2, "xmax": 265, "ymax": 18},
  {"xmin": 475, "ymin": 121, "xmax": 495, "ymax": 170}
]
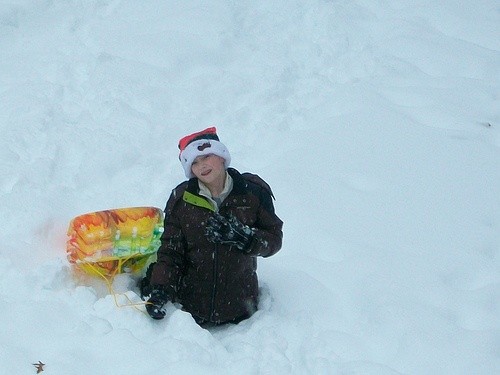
[{"xmin": 138, "ymin": 128, "xmax": 283, "ymax": 329}]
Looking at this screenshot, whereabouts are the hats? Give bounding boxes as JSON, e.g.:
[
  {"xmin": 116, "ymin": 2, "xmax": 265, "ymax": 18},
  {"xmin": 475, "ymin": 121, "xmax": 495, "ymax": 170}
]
[{"xmin": 178, "ymin": 127, "xmax": 231, "ymax": 179}]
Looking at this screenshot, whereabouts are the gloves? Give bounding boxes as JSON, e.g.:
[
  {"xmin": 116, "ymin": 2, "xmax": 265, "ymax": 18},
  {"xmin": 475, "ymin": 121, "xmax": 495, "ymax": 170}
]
[
  {"xmin": 204, "ymin": 211, "xmax": 256, "ymax": 253},
  {"xmin": 145, "ymin": 285, "xmax": 171, "ymax": 319}
]
